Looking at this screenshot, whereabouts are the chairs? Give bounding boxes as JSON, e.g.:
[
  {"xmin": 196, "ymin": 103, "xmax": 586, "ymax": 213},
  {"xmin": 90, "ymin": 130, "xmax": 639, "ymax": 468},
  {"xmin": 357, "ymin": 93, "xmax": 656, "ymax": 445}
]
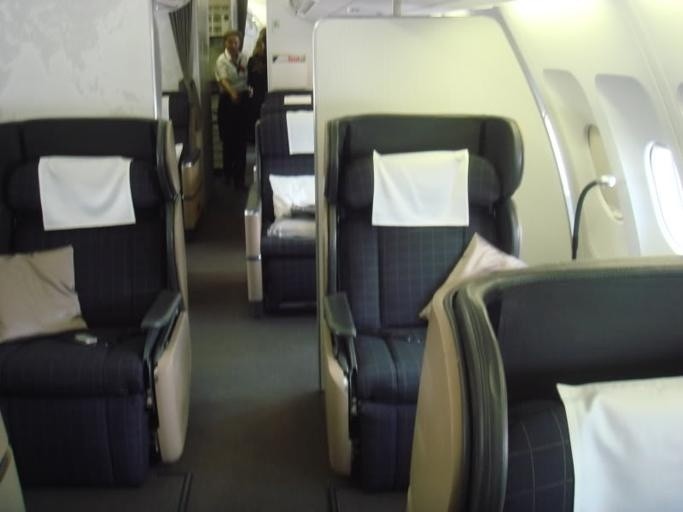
[
  {"xmin": 323, "ymin": 113, "xmax": 525, "ymax": 494},
  {"xmin": 0, "ymin": 115, "xmax": 194, "ymax": 486},
  {"xmin": 160, "ymin": 89, "xmax": 204, "ymax": 235},
  {"xmin": 239, "ymin": 88, "xmax": 317, "ymax": 320},
  {"xmin": 453, "ymin": 257, "xmax": 682, "ymax": 510}
]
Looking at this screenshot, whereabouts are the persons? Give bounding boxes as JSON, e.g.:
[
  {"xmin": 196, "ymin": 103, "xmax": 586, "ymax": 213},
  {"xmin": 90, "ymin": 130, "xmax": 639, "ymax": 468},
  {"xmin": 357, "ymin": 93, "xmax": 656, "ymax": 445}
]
[
  {"xmin": 215, "ymin": 30, "xmax": 252, "ymax": 193},
  {"xmin": 246, "ymin": 27, "xmax": 267, "ymax": 144}
]
